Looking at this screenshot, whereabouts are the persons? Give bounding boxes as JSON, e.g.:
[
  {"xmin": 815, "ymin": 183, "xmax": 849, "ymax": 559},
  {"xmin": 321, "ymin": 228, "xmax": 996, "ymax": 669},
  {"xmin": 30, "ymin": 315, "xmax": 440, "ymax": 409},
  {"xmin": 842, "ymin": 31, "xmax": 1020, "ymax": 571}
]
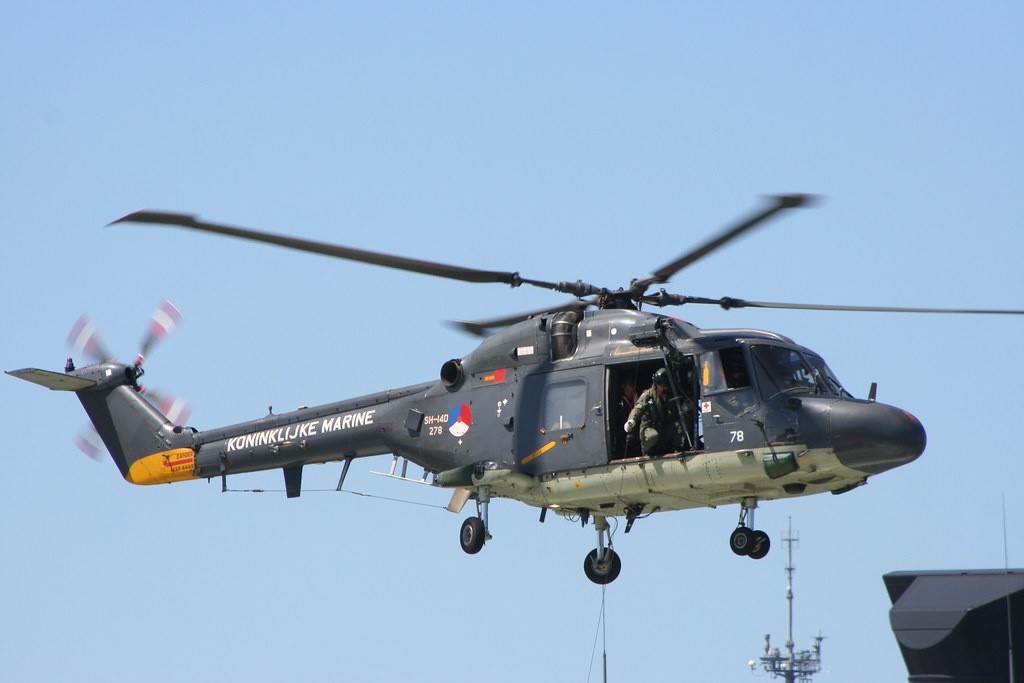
[{"xmin": 624, "ymin": 368, "xmax": 694, "ymax": 456}]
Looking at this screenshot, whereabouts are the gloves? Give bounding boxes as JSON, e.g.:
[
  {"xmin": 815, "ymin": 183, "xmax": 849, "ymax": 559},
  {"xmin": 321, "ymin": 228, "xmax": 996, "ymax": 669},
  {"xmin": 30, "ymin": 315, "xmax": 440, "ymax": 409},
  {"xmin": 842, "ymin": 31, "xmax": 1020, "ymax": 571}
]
[{"xmin": 623, "ymin": 418, "xmax": 636, "ymax": 432}]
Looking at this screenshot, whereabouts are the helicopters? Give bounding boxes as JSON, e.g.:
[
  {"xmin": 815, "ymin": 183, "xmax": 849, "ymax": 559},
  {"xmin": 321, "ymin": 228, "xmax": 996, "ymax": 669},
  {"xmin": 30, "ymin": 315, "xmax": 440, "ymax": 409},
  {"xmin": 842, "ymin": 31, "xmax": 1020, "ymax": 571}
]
[{"xmin": 0, "ymin": 189, "xmax": 1024, "ymax": 586}]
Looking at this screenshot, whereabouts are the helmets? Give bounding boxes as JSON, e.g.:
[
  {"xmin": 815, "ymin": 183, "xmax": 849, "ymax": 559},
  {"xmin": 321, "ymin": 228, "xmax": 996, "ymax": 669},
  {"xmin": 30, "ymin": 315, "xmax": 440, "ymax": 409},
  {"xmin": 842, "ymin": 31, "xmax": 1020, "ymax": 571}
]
[{"xmin": 652, "ymin": 368, "xmax": 668, "ymax": 384}]
